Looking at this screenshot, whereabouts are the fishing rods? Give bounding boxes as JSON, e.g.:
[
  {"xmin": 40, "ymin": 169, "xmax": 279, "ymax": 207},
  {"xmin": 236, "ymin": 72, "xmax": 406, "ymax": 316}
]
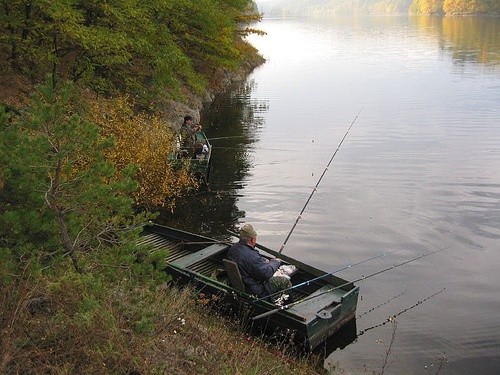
[
  {"xmin": 356, "ymin": 288, "xmax": 445, "ymax": 336},
  {"xmin": 250, "ymin": 247, "xmax": 447, "ymax": 320},
  {"xmin": 199, "ymin": 53, "xmax": 251, "ymax": 123},
  {"xmin": 250, "ymin": 245, "xmax": 407, "ymax": 303},
  {"xmin": 278, "ymin": 109, "xmax": 362, "ymax": 251},
  {"xmin": 183, "ymin": 144, "xmax": 290, "ymax": 151},
  {"xmin": 356, "ymin": 292, "xmax": 408, "ymax": 319}
]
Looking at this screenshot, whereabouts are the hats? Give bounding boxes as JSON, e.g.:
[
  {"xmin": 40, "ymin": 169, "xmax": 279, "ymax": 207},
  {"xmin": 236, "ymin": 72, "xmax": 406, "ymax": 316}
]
[{"xmin": 239, "ymin": 224, "xmax": 257, "ymax": 237}]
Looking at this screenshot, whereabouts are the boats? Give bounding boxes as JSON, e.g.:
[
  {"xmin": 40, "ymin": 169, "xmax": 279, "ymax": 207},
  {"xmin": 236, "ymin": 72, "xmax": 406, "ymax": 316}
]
[
  {"xmin": 102, "ymin": 223, "xmax": 360, "ymax": 357},
  {"xmin": 166, "ymin": 131, "xmax": 212, "ymax": 176}
]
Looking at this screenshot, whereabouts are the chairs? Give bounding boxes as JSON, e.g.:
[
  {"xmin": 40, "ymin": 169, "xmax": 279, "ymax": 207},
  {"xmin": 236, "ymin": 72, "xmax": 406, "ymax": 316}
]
[{"xmin": 222, "ymin": 258, "xmax": 246, "ymax": 293}]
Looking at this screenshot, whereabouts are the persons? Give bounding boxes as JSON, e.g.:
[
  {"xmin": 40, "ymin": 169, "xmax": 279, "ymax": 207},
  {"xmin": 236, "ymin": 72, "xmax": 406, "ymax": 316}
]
[
  {"xmin": 225, "ymin": 223, "xmax": 294, "ymax": 308},
  {"xmin": 180, "ymin": 115, "xmax": 202, "ymax": 145}
]
